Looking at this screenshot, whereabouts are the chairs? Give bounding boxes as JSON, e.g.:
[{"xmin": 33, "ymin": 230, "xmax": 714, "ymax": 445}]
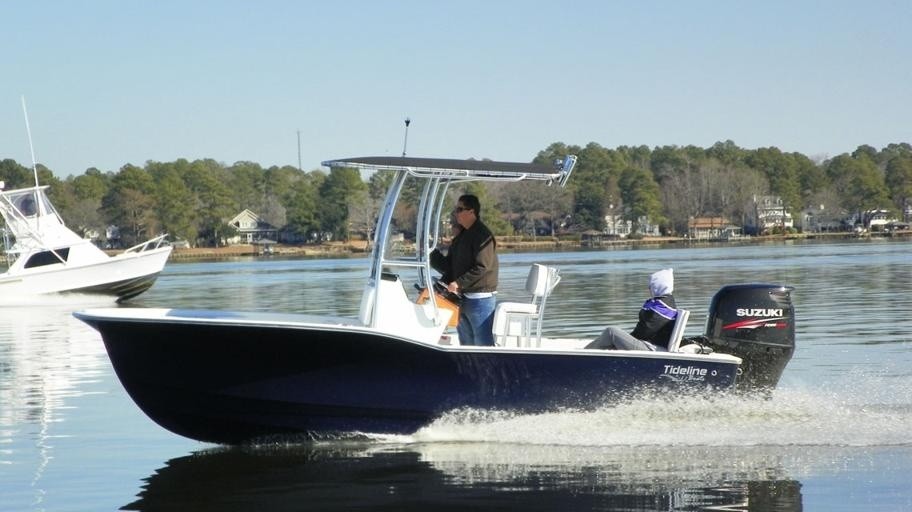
[
  {"xmin": 667, "ymin": 309, "xmax": 691, "ymax": 353},
  {"xmin": 493, "ymin": 264, "xmax": 551, "ymax": 350}
]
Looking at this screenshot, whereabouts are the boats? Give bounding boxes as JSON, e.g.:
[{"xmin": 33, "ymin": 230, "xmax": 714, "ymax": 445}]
[
  {"xmin": 0, "ymin": 95, "xmax": 170, "ymax": 303},
  {"xmin": 71, "ymin": 150, "xmax": 793, "ymax": 443}
]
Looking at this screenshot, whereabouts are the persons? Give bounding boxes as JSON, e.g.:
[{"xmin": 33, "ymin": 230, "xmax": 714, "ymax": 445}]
[
  {"xmin": 438, "ymin": 195, "xmax": 498, "ymax": 347},
  {"xmin": 397, "ymin": 211, "xmax": 466, "ymax": 278},
  {"xmin": 583, "ymin": 268, "xmax": 679, "ymax": 352}
]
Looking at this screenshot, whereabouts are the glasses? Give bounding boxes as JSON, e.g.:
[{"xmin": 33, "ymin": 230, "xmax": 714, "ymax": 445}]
[{"xmin": 456, "ymin": 207, "xmax": 472, "ymax": 212}]
